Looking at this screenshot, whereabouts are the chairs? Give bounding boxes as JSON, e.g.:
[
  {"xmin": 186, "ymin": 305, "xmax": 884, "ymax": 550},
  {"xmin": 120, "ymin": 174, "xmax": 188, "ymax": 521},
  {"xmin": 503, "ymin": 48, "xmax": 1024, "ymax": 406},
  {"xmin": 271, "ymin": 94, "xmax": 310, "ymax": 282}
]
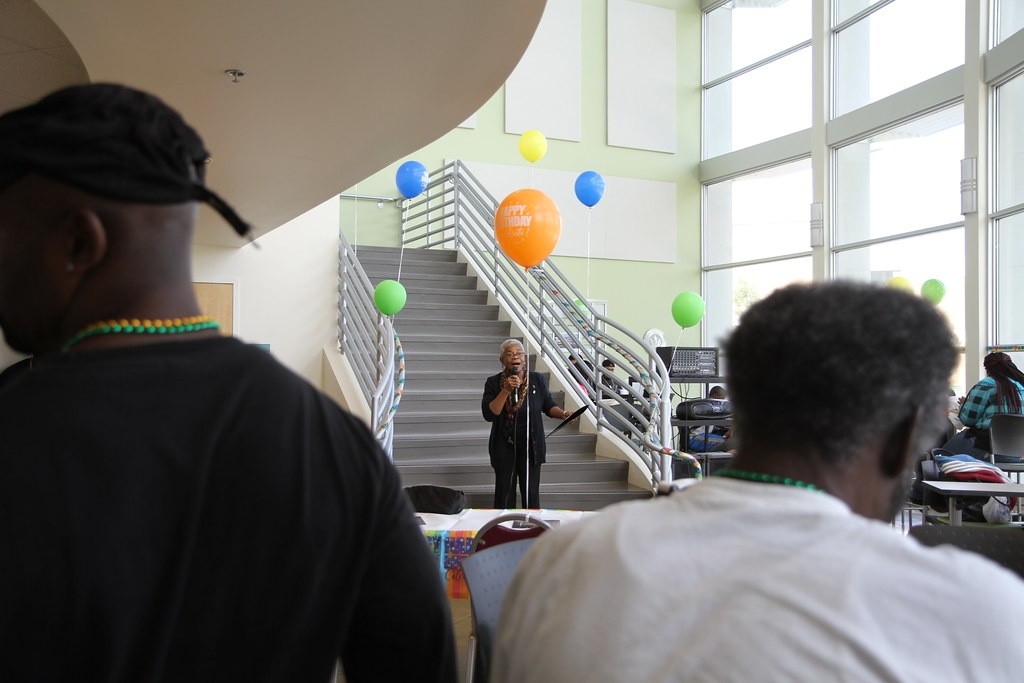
[
  {"xmin": 986, "ymin": 414, "xmax": 1024, "ymax": 482},
  {"xmin": 911, "ymin": 524, "xmax": 1023, "ymax": 579}
]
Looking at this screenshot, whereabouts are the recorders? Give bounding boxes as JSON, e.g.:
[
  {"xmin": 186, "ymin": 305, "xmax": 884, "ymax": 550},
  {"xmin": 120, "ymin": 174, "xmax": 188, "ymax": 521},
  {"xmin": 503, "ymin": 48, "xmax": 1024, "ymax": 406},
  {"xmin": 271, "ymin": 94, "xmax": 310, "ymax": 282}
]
[{"xmin": 676, "ymin": 398, "xmax": 733, "ymax": 419}]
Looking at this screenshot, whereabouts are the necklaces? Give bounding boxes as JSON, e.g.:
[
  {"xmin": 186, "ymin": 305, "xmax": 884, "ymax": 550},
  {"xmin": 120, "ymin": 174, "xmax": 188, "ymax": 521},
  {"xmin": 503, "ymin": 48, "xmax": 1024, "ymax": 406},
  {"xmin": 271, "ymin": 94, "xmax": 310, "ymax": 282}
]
[
  {"xmin": 711, "ymin": 468, "xmax": 825, "ymax": 494},
  {"xmin": 602, "ymin": 377, "xmax": 614, "ymax": 390},
  {"xmin": 62, "ymin": 314, "xmax": 218, "ymax": 350}
]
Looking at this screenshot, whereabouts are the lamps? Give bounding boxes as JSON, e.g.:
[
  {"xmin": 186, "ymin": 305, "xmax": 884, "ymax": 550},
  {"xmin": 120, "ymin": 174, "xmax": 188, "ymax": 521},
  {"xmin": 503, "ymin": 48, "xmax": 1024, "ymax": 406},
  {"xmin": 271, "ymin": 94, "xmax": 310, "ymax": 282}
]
[
  {"xmin": 811, "ymin": 202, "xmax": 824, "ymax": 245},
  {"xmin": 960, "ymin": 157, "xmax": 975, "ymax": 214}
]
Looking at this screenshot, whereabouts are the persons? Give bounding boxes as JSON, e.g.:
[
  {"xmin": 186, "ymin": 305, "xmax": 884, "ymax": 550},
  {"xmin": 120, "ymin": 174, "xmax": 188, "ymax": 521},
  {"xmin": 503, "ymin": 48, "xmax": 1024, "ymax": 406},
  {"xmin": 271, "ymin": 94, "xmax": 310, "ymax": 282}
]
[
  {"xmin": 0, "ymin": 83, "xmax": 458, "ymax": 683},
  {"xmin": 594, "ymin": 360, "xmax": 622, "ymax": 399},
  {"xmin": 492, "ymin": 279, "xmax": 1024, "ymax": 683},
  {"xmin": 688, "ymin": 386, "xmax": 727, "ymax": 451},
  {"xmin": 481, "ymin": 338, "xmax": 579, "ymax": 509},
  {"xmin": 942, "ymin": 353, "xmax": 1024, "ymax": 463},
  {"xmin": 569, "ymin": 360, "xmax": 593, "ymax": 397}
]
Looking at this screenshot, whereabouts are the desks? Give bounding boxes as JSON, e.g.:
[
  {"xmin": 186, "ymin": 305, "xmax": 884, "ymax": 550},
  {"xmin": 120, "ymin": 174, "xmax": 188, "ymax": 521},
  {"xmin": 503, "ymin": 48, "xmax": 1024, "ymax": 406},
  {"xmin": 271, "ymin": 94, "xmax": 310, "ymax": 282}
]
[
  {"xmin": 920, "ymin": 481, "xmax": 1023, "ymax": 526},
  {"xmin": 670, "ymin": 417, "xmax": 735, "ymax": 477},
  {"xmin": 627, "ymin": 377, "xmax": 728, "ymax": 440}
]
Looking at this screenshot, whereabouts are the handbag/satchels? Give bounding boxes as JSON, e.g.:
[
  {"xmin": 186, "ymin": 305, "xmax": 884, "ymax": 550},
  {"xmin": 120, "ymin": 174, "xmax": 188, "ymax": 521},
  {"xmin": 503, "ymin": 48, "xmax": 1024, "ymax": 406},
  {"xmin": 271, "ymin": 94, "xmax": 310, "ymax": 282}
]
[{"xmin": 930, "ymin": 448, "xmax": 1011, "ymax": 520}]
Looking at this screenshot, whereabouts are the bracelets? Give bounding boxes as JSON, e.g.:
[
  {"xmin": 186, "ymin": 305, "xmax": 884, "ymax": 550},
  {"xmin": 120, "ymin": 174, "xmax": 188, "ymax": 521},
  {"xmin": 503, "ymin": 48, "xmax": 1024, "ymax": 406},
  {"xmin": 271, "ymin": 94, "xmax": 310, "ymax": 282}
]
[{"xmin": 563, "ymin": 411, "xmax": 566, "ymax": 415}]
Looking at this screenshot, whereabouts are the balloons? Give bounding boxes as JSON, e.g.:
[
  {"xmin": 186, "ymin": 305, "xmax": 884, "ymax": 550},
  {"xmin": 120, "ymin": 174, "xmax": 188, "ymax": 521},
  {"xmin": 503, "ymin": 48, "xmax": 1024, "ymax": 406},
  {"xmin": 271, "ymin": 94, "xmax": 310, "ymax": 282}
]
[
  {"xmin": 921, "ymin": 279, "xmax": 945, "ymax": 305},
  {"xmin": 886, "ymin": 276, "xmax": 913, "ymax": 291},
  {"xmin": 519, "ymin": 129, "xmax": 547, "ymax": 163},
  {"xmin": 396, "ymin": 160, "xmax": 429, "ymax": 200},
  {"xmin": 574, "ymin": 171, "xmax": 606, "ymax": 208},
  {"xmin": 373, "ymin": 279, "xmax": 406, "ymax": 316},
  {"xmin": 672, "ymin": 291, "xmax": 705, "ymax": 327},
  {"xmin": 494, "ymin": 188, "xmax": 561, "ymax": 268}
]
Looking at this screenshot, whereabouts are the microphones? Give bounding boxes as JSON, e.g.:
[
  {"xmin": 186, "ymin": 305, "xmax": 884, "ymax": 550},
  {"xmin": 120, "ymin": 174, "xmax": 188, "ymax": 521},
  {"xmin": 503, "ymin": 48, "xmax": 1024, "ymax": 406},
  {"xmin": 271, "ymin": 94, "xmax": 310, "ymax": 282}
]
[{"xmin": 511, "ymin": 367, "xmax": 519, "ymax": 406}]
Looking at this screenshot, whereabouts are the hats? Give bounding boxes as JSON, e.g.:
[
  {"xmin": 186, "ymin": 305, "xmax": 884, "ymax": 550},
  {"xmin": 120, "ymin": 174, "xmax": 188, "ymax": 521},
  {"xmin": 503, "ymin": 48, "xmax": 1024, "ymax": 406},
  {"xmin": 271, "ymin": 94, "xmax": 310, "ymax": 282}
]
[
  {"xmin": 603, "ymin": 360, "xmax": 615, "ymax": 367},
  {"xmin": 0, "ymin": 82, "xmax": 248, "ymax": 234}
]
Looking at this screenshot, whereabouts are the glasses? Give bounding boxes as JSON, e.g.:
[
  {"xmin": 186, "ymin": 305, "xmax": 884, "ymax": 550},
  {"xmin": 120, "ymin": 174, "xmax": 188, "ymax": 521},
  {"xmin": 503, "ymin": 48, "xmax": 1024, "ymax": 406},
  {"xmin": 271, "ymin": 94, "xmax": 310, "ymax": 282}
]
[{"xmin": 506, "ymin": 352, "xmax": 526, "ymax": 356}]
